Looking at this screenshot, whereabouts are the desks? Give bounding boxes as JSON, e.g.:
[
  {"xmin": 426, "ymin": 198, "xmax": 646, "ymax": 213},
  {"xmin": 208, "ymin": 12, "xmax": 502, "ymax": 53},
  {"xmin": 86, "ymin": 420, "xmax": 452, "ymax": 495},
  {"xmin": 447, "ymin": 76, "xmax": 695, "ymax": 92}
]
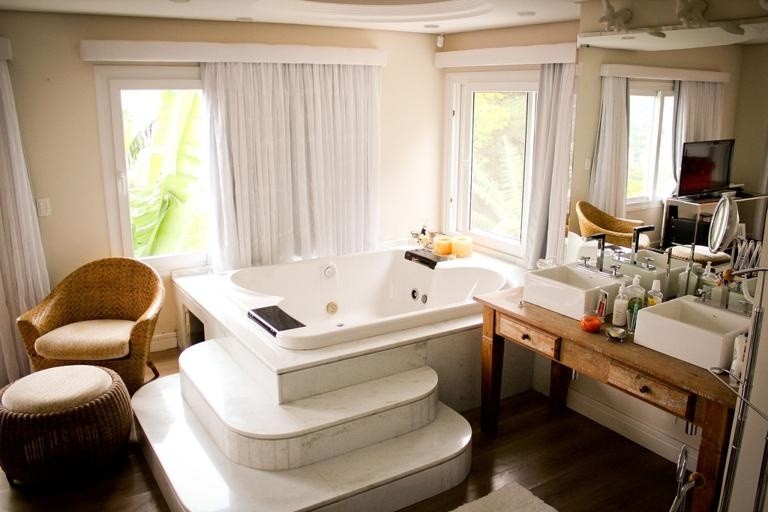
[
  {"xmin": 474, "ymin": 288, "xmax": 740, "ymax": 512},
  {"xmin": 659, "ymin": 193, "xmax": 768, "ymax": 253}
]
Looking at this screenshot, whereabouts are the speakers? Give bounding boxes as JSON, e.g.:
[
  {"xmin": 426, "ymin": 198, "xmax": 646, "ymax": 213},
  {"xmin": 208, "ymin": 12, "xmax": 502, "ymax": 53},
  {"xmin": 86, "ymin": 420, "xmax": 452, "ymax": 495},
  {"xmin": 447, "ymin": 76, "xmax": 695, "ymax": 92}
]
[{"xmin": 669, "ymin": 206, "xmax": 678, "ymax": 219}]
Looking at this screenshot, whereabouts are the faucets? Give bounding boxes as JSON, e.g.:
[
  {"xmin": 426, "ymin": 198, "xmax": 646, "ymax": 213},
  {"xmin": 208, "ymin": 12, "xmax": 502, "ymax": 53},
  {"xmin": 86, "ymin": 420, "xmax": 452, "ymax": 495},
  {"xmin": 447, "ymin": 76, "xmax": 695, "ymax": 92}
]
[
  {"xmin": 631, "ymin": 223, "xmax": 657, "ymax": 264},
  {"xmin": 582, "ymin": 232, "xmax": 606, "ymax": 271}
]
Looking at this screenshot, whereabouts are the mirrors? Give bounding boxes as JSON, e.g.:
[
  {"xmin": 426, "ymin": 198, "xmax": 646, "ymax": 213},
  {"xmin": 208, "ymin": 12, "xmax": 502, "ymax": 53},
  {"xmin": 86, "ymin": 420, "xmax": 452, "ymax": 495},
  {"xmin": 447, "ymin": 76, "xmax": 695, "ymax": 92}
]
[{"xmin": 564, "ymin": 21, "xmax": 767, "ymax": 269}]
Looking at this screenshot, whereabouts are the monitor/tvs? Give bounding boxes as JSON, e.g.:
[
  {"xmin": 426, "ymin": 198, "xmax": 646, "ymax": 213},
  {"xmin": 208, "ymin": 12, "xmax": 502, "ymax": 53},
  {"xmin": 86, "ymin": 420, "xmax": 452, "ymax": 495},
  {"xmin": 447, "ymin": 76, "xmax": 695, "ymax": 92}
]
[{"xmin": 678, "ymin": 140, "xmax": 735, "ymax": 203}]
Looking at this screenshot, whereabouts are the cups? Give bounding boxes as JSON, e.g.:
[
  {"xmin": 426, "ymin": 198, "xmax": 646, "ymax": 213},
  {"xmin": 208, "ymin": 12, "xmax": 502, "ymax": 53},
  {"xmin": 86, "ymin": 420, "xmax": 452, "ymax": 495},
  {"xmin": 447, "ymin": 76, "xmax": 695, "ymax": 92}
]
[
  {"xmin": 452, "ymin": 234, "xmax": 473, "ymax": 259},
  {"xmin": 431, "ymin": 236, "xmax": 453, "ymax": 256},
  {"xmin": 579, "ymin": 311, "xmax": 601, "ymax": 333}
]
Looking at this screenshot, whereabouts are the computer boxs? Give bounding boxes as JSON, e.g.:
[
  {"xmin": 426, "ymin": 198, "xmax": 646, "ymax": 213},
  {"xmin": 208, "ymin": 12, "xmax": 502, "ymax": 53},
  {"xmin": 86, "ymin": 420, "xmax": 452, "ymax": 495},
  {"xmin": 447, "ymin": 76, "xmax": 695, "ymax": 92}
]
[{"xmin": 663, "ymin": 218, "xmax": 710, "ymax": 249}]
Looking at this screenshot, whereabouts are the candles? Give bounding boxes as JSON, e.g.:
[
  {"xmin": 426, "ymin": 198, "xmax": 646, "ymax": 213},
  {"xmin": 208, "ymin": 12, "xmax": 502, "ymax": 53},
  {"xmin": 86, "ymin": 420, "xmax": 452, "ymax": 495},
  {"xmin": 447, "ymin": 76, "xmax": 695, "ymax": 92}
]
[
  {"xmin": 454, "ymin": 235, "xmax": 471, "ymax": 258},
  {"xmin": 433, "ymin": 235, "xmax": 452, "ymax": 256}
]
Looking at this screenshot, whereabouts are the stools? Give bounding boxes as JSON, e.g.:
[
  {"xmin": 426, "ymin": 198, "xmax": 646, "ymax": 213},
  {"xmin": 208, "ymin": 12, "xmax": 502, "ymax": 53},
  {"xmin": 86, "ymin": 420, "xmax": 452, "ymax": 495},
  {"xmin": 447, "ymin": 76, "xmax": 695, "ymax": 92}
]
[
  {"xmin": 0, "ymin": 364, "xmax": 134, "ymax": 503},
  {"xmin": 662, "ymin": 243, "xmax": 731, "ymax": 272}
]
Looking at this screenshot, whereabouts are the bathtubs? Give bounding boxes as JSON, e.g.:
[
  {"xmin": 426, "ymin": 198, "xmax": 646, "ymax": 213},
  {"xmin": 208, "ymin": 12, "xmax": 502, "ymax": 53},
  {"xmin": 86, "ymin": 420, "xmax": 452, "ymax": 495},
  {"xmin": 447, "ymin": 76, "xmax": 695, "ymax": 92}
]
[{"xmin": 225, "ymin": 240, "xmax": 506, "ymax": 352}]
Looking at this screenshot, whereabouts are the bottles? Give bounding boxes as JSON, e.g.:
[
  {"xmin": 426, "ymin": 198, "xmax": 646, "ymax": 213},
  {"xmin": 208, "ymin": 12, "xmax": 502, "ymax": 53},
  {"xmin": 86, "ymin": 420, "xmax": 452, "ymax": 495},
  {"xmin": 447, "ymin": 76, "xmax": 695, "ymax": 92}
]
[
  {"xmin": 646, "ymin": 279, "xmax": 663, "ymax": 306},
  {"xmin": 676, "ymin": 261, "xmax": 720, "ymax": 299}
]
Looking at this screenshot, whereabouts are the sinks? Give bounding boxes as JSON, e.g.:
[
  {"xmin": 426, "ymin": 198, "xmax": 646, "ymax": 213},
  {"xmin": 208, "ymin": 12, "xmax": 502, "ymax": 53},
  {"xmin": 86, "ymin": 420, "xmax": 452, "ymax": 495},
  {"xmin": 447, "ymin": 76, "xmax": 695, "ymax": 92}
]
[
  {"xmin": 630, "ymin": 292, "xmax": 751, "ymax": 373},
  {"xmin": 522, "ymin": 261, "xmax": 633, "ymax": 323},
  {"xmin": 601, "ymin": 247, "xmax": 703, "ymax": 300}
]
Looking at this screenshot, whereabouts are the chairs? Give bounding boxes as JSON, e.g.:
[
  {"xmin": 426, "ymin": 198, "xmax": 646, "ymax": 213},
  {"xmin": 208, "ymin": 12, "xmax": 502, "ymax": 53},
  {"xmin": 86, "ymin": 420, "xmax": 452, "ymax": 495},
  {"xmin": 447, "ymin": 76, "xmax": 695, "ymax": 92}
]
[
  {"xmin": 15, "ymin": 258, "xmax": 166, "ymax": 399},
  {"xmin": 574, "ymin": 199, "xmax": 647, "ymax": 250}
]
